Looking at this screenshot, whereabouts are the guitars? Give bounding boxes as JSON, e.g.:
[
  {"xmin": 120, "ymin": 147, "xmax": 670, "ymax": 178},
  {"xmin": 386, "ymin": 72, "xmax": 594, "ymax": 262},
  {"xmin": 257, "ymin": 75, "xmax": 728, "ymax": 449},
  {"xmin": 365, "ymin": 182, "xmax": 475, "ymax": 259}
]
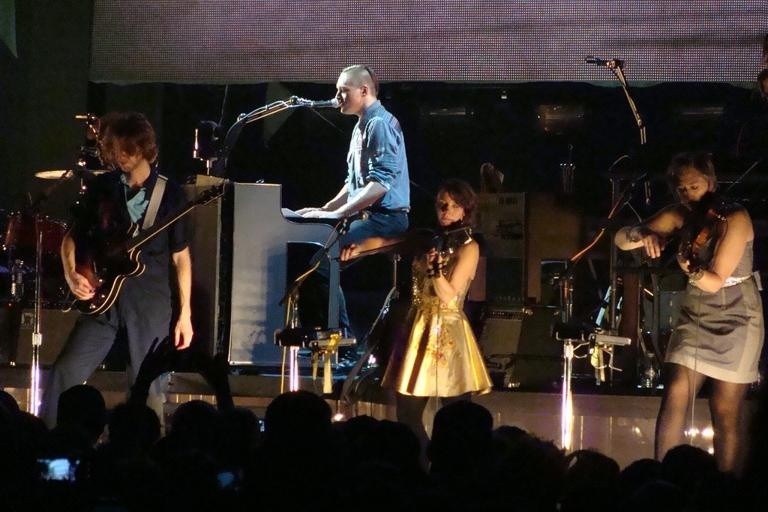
[{"xmin": 69, "ymin": 179, "xmax": 226, "ymax": 316}]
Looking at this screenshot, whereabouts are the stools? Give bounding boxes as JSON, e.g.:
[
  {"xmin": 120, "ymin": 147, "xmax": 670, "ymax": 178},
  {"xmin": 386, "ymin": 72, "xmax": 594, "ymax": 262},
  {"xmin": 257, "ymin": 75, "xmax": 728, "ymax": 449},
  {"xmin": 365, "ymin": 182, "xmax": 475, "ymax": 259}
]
[{"xmin": 338, "ymin": 228, "xmax": 435, "ymax": 405}]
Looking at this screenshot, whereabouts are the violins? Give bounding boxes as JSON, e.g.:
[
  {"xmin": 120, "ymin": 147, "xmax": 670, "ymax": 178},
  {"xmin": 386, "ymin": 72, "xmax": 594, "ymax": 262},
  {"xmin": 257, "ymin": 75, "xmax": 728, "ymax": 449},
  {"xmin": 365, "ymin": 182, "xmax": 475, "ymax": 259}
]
[
  {"xmin": 410, "ymin": 219, "xmax": 472, "ymax": 278},
  {"xmin": 666, "ymin": 191, "xmax": 730, "ymax": 273}
]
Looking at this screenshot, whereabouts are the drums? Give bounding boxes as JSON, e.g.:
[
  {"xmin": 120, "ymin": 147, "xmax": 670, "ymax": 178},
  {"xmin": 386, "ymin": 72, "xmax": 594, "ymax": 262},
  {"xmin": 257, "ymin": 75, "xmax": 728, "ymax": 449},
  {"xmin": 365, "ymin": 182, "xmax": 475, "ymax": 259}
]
[{"xmin": 4, "ymin": 212, "xmax": 67, "ymax": 263}]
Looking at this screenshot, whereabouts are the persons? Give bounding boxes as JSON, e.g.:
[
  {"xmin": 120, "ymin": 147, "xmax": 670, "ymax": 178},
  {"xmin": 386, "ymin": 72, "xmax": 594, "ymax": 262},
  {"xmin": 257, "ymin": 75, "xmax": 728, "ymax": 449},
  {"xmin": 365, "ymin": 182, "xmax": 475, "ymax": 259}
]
[
  {"xmin": 290, "ymin": 63, "xmax": 412, "ymax": 347},
  {"xmin": 44, "ymin": 118, "xmax": 117, "ymax": 197},
  {"xmin": 38, "ymin": 111, "xmax": 194, "ymax": 451},
  {"xmin": 338, "ymin": 178, "xmax": 494, "ymax": 476},
  {"xmin": 613, "ymin": 151, "xmax": 766, "ymax": 475}
]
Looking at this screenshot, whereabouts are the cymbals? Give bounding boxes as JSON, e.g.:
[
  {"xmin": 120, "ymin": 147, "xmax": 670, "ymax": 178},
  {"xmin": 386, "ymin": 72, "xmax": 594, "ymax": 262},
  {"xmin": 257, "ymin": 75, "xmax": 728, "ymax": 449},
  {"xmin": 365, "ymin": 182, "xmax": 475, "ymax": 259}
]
[{"xmin": 36, "ymin": 170, "xmax": 107, "ymax": 178}]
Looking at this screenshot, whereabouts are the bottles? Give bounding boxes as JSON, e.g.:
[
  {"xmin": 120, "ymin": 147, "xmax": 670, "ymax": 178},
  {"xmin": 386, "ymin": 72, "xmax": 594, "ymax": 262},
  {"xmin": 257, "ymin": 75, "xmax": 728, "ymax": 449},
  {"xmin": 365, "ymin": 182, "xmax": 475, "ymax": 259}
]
[{"xmin": 11, "ymin": 259, "xmax": 25, "ymax": 296}]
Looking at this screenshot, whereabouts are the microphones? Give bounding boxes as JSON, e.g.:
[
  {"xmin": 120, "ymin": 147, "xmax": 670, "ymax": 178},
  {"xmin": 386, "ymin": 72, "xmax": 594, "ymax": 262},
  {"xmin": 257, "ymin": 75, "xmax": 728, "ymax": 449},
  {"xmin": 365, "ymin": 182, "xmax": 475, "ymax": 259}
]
[
  {"xmin": 75, "ymin": 114, "xmax": 97, "ymax": 122},
  {"xmin": 586, "ymin": 56, "xmax": 625, "ymax": 67},
  {"xmin": 310, "ymin": 98, "xmax": 339, "ymax": 109}
]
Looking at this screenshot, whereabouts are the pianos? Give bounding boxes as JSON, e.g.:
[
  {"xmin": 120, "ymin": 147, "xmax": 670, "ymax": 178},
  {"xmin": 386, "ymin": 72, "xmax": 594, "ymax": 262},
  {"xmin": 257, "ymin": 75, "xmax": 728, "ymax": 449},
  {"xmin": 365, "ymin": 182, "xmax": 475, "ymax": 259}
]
[{"xmin": 181, "ymin": 175, "xmax": 341, "ymax": 372}]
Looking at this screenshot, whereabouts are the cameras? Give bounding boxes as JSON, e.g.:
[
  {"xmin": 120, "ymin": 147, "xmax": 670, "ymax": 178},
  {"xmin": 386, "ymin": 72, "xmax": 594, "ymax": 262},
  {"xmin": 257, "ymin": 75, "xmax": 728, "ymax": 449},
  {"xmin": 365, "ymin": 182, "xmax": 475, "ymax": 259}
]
[{"xmin": 38, "ymin": 455, "xmax": 83, "ymax": 481}]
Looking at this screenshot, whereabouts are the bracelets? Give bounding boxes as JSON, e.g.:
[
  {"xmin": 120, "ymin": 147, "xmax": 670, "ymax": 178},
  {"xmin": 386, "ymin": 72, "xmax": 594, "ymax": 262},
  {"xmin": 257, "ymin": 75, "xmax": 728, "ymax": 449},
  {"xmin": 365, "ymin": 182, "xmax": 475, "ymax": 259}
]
[{"xmin": 690, "ymin": 269, "xmax": 703, "ymax": 281}]
